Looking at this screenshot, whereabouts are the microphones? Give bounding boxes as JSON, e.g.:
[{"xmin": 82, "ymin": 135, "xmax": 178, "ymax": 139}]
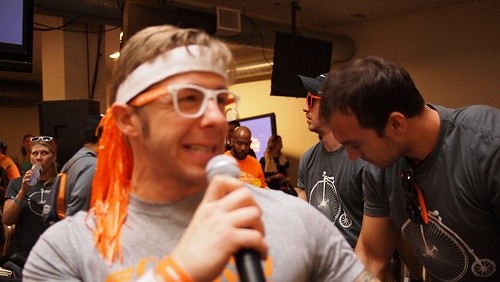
[{"xmin": 205, "ymin": 153, "xmax": 265, "ymax": 282}]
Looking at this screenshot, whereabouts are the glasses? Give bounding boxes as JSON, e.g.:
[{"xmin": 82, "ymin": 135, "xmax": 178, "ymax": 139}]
[
  {"xmin": 400, "ymin": 169, "xmax": 428, "ymax": 225},
  {"xmin": 306, "ymin": 94, "xmax": 321, "ymax": 107},
  {"xmin": 125, "ymin": 83, "xmax": 240, "ymax": 122},
  {"xmin": 30, "ymin": 136, "xmax": 53, "ymax": 143}
]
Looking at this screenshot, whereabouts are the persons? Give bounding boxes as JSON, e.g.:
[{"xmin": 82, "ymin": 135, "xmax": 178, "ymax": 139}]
[
  {"xmin": 21, "ymin": 23, "xmax": 382, "ymax": 282},
  {"xmin": 317, "ymin": 54, "xmax": 500, "ymax": 282},
  {"xmin": 258, "ymin": 135, "xmax": 299, "ymax": 198},
  {"xmin": 294, "ymin": 72, "xmax": 423, "ymax": 281},
  {"xmin": 225, "ymin": 119, "xmax": 257, "ymax": 158},
  {"xmin": 224, "ymin": 126, "xmax": 269, "ymax": 189},
  {"xmin": 0, "ymin": 115, "xmax": 106, "ymax": 282}
]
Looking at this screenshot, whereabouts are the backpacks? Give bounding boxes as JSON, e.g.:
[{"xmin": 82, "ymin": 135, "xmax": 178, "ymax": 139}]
[{"xmin": 42, "ymin": 153, "xmax": 95, "ymax": 227}]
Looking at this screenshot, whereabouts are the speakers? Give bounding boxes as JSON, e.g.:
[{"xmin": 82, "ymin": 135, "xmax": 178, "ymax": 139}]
[
  {"xmin": 39, "ymin": 99, "xmax": 101, "ymax": 168},
  {"xmin": 269, "ymin": 31, "xmax": 333, "ymax": 97}
]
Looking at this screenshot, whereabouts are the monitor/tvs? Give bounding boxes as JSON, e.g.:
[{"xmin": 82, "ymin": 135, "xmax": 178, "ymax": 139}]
[{"xmin": 0, "ymin": 0, "xmax": 35, "ymax": 72}]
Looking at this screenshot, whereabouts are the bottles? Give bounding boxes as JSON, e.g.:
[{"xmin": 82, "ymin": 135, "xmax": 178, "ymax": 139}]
[{"xmin": 26, "ymin": 163, "xmax": 43, "ymax": 186}]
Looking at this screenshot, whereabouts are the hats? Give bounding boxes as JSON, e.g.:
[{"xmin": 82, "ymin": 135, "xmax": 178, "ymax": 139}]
[{"xmin": 298, "ymin": 73, "xmax": 326, "ymax": 96}]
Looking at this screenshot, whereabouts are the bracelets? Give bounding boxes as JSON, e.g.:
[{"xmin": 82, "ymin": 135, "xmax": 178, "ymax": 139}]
[
  {"xmin": 156, "ymin": 256, "xmax": 192, "ymax": 282},
  {"xmin": 15, "ymin": 197, "xmax": 22, "ymax": 205}
]
